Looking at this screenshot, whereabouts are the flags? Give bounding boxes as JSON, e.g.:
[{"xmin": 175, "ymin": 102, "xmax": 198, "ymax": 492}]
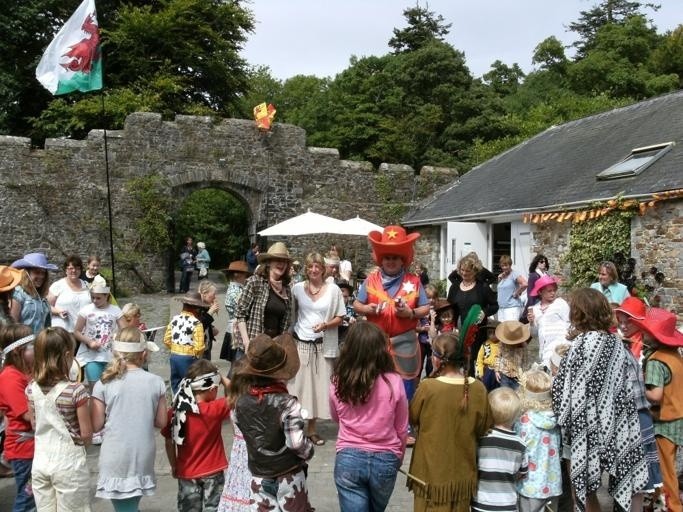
[{"xmin": 35, "ymin": 0, "xmax": 105, "ymax": 96}]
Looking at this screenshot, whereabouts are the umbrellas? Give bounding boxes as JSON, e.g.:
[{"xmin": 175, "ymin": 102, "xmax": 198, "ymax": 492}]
[
  {"xmin": 257, "ymin": 208, "xmax": 347, "ymax": 252},
  {"xmin": 342, "ymin": 214, "xmax": 384, "ymax": 266}
]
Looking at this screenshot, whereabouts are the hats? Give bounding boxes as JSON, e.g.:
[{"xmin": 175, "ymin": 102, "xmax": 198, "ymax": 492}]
[
  {"xmin": 10, "ymin": 253, "xmax": 58, "ymax": 271},
  {"xmin": 0, "ymin": 265, "xmax": 22, "ymax": 293},
  {"xmin": 435, "ymin": 300, "xmax": 451, "ymax": 310},
  {"xmin": 367, "ymin": 226, "xmax": 421, "ymax": 273},
  {"xmin": 88, "ymin": 274, "xmax": 110, "ymax": 294},
  {"xmin": 256, "ymin": 241, "xmax": 296, "ymax": 264},
  {"xmin": 629, "ymin": 307, "xmax": 683, "ymax": 347},
  {"xmin": 173, "ymin": 289, "xmax": 210, "ymax": 308},
  {"xmin": 495, "ymin": 320, "xmax": 531, "ymax": 345},
  {"xmin": 111, "ymin": 329, "xmax": 159, "ymax": 353},
  {"xmin": 221, "ymin": 261, "xmax": 253, "ymax": 275},
  {"xmin": 539, "ymin": 260, "xmax": 547, "ymax": 264},
  {"xmin": 612, "ymin": 296, "xmax": 647, "ymax": 320},
  {"xmin": 235, "ymin": 333, "xmax": 301, "ymax": 380},
  {"xmin": 530, "ymin": 275, "xmax": 559, "ymax": 297},
  {"xmin": 335, "ymin": 279, "xmax": 354, "ymax": 294}
]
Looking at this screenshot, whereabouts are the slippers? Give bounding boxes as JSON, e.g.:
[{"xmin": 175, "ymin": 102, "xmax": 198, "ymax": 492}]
[{"xmin": 307, "ymin": 434, "xmax": 325, "ymax": 446}]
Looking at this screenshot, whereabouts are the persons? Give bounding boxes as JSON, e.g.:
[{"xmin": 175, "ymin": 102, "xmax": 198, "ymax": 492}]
[
  {"xmin": 91, "ymin": 285, "xmax": 233, "ymax": 512},
  {"xmin": 0, "ymin": 253, "xmax": 126, "ymax": 512},
  {"xmin": 220, "ymin": 226, "xmax": 430, "ymax": 512},
  {"xmin": 178, "ymin": 237, "xmax": 211, "ymax": 291},
  {"xmin": 406, "ymin": 253, "xmax": 683, "ymax": 512}
]
